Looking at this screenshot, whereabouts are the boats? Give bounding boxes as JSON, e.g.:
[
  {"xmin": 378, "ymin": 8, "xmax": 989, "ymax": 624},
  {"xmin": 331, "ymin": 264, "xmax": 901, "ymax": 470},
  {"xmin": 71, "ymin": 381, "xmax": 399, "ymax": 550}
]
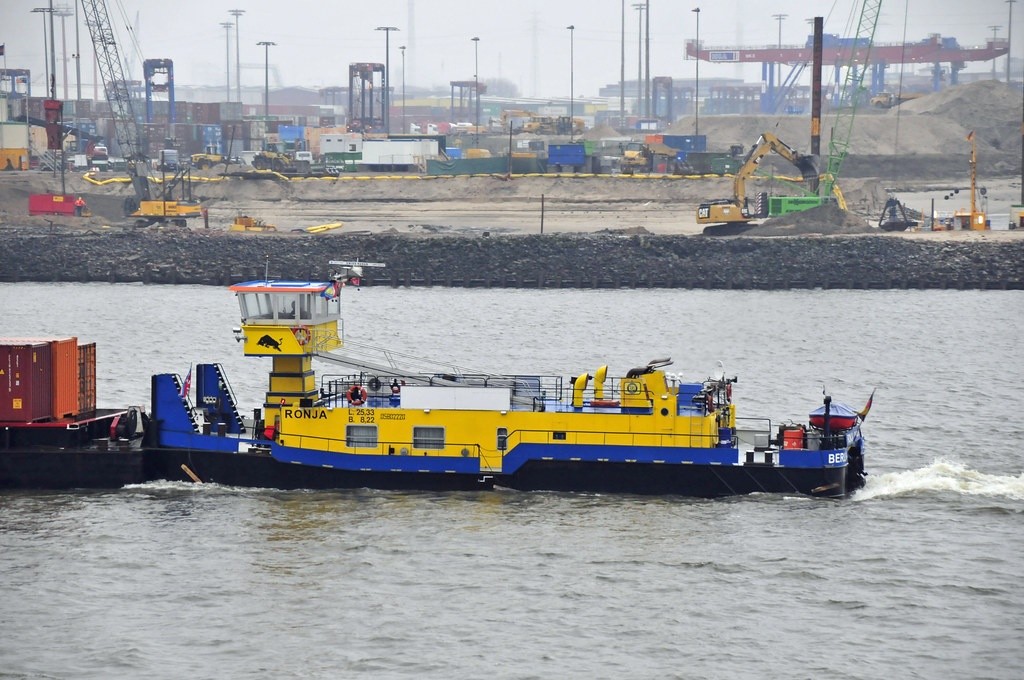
[{"xmin": 0, "ymin": 254, "xmax": 877, "ymax": 500}]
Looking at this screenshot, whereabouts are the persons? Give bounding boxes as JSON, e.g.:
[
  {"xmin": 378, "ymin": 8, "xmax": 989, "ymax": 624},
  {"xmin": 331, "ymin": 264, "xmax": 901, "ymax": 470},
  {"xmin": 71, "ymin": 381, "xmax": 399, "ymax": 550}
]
[
  {"xmin": 74, "ymin": 197, "xmax": 86, "ymax": 212},
  {"xmin": 291, "ymin": 301, "xmax": 303, "ymax": 319}
]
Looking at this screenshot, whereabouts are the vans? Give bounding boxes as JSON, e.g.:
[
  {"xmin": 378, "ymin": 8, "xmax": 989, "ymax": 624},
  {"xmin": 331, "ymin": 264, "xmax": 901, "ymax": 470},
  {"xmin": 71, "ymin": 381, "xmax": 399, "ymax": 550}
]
[{"xmin": 295, "ymin": 151, "xmax": 315, "ymax": 165}]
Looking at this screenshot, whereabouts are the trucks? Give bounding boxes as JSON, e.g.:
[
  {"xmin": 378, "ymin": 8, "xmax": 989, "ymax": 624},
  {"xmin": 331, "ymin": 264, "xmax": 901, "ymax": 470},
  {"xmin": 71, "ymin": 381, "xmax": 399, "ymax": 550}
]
[
  {"xmin": 620, "ymin": 134, "xmax": 705, "ymax": 175},
  {"xmin": 757, "ymin": 0, "xmax": 882, "ymax": 220},
  {"xmin": 158, "ymin": 150, "xmax": 179, "ymax": 172}
]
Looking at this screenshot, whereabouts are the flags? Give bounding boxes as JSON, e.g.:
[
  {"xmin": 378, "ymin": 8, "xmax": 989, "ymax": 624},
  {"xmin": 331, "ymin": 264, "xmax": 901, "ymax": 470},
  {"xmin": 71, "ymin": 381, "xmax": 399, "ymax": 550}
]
[
  {"xmin": 178, "ymin": 370, "xmax": 191, "ymax": 397},
  {"xmin": 854, "ymin": 394, "xmax": 874, "ymax": 421},
  {"xmin": 319, "ymin": 281, "xmax": 340, "ymax": 301}
]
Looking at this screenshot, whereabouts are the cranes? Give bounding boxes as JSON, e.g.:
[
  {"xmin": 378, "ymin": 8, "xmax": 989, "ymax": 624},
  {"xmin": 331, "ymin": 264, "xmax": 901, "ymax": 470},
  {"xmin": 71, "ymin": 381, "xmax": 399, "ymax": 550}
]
[{"xmin": 82, "ymin": 0, "xmax": 201, "ymax": 231}]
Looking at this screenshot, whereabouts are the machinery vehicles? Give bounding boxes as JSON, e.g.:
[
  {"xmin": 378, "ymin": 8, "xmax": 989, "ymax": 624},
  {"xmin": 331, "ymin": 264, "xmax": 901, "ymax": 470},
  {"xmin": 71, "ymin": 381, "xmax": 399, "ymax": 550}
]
[
  {"xmin": 869, "ymin": 91, "xmax": 925, "ymax": 109},
  {"xmin": 251, "ymin": 143, "xmax": 297, "ymax": 173},
  {"xmin": 696, "ymin": 133, "xmax": 821, "ymax": 236},
  {"xmin": 522, "ymin": 113, "xmax": 585, "ymax": 136},
  {"xmin": 190, "ymin": 141, "xmax": 246, "ymax": 171}
]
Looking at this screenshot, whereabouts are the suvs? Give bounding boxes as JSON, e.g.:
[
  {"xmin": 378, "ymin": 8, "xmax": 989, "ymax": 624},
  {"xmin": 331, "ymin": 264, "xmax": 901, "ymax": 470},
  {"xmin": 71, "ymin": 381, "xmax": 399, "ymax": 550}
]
[{"xmin": 91, "ymin": 146, "xmax": 107, "ymax": 161}]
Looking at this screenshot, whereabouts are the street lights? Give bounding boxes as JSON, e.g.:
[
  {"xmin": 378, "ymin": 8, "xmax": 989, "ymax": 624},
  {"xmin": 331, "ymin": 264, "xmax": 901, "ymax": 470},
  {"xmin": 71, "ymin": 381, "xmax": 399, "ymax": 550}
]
[
  {"xmin": 692, "ymin": 7, "xmax": 700, "ymax": 134},
  {"xmin": 399, "ymin": 46, "xmax": 406, "ymax": 133},
  {"xmin": 256, "ymin": 41, "xmax": 278, "ymax": 120},
  {"xmin": 988, "ymin": 25, "xmax": 1004, "ymax": 80},
  {"xmin": 772, "ymin": 14, "xmax": 789, "ymax": 87},
  {"xmin": 472, "ymin": 37, "xmax": 479, "ymax": 142},
  {"xmin": 1006, "ymin": 0, "xmax": 1017, "ymax": 82},
  {"xmin": 374, "ymin": 27, "xmax": 400, "ymax": 132},
  {"xmin": 567, "ymin": 25, "xmax": 576, "ymax": 143},
  {"xmin": 219, "ymin": 22, "xmax": 235, "ymax": 102},
  {"xmin": 228, "ymin": 8, "xmax": 246, "ymax": 102}
]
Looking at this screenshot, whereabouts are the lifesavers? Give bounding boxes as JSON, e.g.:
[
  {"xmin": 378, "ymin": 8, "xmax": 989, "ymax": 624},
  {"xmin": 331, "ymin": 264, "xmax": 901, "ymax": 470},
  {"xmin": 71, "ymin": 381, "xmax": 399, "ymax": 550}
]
[
  {"xmin": 728, "ymin": 383, "xmax": 732, "ymax": 397},
  {"xmin": 291, "ymin": 324, "xmax": 312, "ymax": 345},
  {"xmin": 708, "ymin": 396, "xmax": 713, "ymax": 411},
  {"xmin": 346, "ymin": 385, "xmax": 367, "ymax": 405},
  {"xmin": 368, "ymin": 377, "xmax": 382, "ymax": 392}
]
